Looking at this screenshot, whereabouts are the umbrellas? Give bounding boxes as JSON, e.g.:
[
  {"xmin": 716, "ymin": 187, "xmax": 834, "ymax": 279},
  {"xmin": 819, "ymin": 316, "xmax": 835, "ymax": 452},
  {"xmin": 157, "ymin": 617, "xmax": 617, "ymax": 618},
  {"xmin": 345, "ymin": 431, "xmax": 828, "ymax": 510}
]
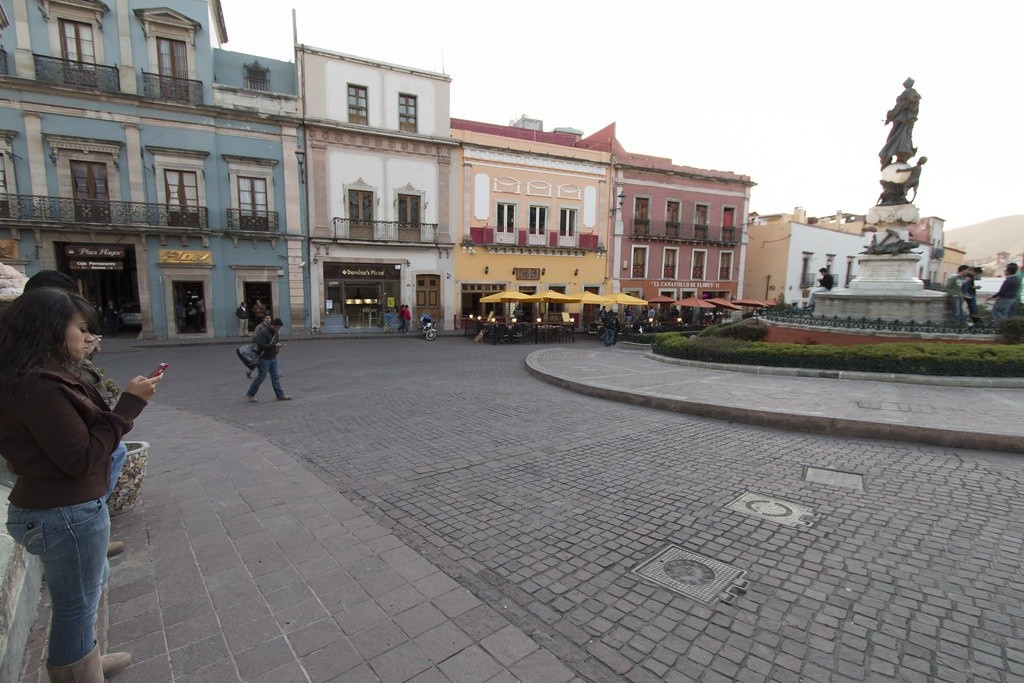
[{"xmin": 479, "ymin": 290, "xmax": 781, "ymax": 322}]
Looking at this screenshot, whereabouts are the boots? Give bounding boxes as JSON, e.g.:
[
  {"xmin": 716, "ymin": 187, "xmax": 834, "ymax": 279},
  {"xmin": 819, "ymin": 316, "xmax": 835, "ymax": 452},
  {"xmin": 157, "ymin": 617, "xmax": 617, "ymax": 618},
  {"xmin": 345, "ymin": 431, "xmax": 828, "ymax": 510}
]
[{"xmin": 46, "ymin": 637, "xmax": 131, "ymax": 683}]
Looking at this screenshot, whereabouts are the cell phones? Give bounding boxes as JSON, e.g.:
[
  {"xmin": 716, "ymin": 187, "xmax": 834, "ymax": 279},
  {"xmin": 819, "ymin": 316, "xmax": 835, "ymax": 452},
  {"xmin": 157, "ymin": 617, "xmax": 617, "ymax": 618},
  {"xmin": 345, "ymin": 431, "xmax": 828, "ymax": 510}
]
[
  {"xmin": 146, "ymin": 363, "xmax": 168, "ymax": 380},
  {"xmin": 281, "ymin": 344, "xmax": 287, "ymax": 346}
]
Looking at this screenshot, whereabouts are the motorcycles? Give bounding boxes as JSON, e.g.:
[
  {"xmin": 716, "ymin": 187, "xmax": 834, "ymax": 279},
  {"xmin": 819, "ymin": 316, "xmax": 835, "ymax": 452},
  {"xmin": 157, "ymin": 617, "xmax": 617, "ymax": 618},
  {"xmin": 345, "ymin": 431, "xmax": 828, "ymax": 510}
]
[{"xmin": 418, "ymin": 313, "xmax": 437, "ymax": 341}]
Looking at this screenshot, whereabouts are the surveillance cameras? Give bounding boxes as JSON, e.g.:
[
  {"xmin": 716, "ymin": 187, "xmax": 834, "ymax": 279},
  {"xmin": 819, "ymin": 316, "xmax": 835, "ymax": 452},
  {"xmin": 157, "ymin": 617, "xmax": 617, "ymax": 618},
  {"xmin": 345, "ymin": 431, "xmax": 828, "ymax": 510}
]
[{"xmin": 299, "ymin": 261, "xmax": 306, "ymax": 267}]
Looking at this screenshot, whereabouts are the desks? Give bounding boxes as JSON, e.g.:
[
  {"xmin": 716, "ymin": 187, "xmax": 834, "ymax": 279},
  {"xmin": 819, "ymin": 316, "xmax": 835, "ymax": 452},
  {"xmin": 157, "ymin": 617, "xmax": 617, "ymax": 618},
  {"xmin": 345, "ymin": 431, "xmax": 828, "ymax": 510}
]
[{"xmin": 362, "ymin": 309, "xmax": 378, "ymax": 322}]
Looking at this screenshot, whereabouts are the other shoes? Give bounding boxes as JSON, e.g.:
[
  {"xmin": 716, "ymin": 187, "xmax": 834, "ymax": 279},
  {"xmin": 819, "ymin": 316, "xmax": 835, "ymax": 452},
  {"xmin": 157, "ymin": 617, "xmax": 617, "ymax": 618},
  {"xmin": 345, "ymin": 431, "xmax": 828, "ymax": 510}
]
[
  {"xmin": 246, "ymin": 368, "xmax": 252, "ymax": 379},
  {"xmin": 277, "ymin": 394, "xmax": 292, "ymax": 400},
  {"xmin": 246, "ymin": 392, "xmax": 257, "ymax": 402},
  {"xmin": 107, "ymin": 541, "xmax": 124, "ymax": 556}
]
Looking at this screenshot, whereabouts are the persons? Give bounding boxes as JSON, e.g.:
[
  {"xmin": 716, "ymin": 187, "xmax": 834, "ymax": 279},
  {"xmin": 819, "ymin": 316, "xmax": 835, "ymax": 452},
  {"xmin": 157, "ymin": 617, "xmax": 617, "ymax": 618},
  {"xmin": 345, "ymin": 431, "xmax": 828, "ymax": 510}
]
[
  {"xmin": 803, "ymin": 268, "xmax": 834, "ymax": 310},
  {"xmin": 253, "ymin": 299, "xmax": 266, "ymax": 327},
  {"xmin": 385, "ymin": 310, "xmax": 396, "ymax": 333},
  {"xmin": 104, "ymin": 297, "xmax": 119, "ymax": 337},
  {"xmin": 0, "ymin": 286, "xmax": 164, "ymax": 683},
  {"xmin": 246, "ymin": 318, "xmax": 292, "ymax": 402},
  {"xmin": 896, "ymin": 156, "xmax": 927, "ymax": 203},
  {"xmin": 24, "ymin": 269, "xmax": 127, "ymax": 557},
  {"xmin": 984, "ymin": 263, "xmax": 1023, "ymax": 320},
  {"xmin": 176, "ymin": 298, "xmax": 205, "ymax": 333},
  {"xmin": 599, "ymin": 306, "xmax": 720, "ymax": 347},
  {"xmin": 513, "ymin": 304, "xmax": 524, "ymax": 323},
  {"xmin": 246, "ymin": 315, "xmax": 282, "ymax": 379},
  {"xmin": 878, "ymin": 77, "xmax": 921, "ymax": 172},
  {"xmin": 397, "ymin": 304, "xmax": 411, "ymax": 334},
  {"xmin": 235, "ymin": 302, "xmax": 251, "ymax": 338},
  {"xmin": 945, "ymin": 264, "xmax": 982, "ymax": 327}
]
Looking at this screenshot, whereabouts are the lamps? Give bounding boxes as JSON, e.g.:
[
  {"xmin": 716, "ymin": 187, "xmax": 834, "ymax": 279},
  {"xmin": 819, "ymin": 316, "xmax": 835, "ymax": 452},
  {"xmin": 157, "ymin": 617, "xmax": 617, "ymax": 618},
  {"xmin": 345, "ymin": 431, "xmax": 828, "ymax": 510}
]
[
  {"xmin": 541, "ymin": 268, "xmax": 546, "ymax": 276},
  {"xmin": 486, "ymin": 266, "xmax": 489, "ymax": 276},
  {"xmin": 611, "ymin": 190, "xmax": 626, "ymax": 214},
  {"xmin": 295, "ymin": 144, "xmax": 306, "ymax": 183},
  {"xmin": 574, "ymin": 269, "xmax": 578, "ymax": 276},
  {"xmin": 739, "ymin": 210, "xmax": 759, "ymax": 227},
  {"xmin": 313, "ymin": 256, "xmax": 318, "ymax": 264},
  {"xmin": 512, "ymin": 267, "xmax": 516, "ymax": 275},
  {"xmin": 407, "ymin": 259, "xmax": 410, "ymax": 266}
]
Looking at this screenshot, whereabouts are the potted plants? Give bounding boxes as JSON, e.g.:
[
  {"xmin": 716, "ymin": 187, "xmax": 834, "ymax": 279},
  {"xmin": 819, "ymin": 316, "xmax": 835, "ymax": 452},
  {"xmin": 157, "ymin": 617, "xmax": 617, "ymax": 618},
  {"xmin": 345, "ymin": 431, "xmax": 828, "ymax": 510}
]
[{"xmin": 94, "ymin": 366, "xmax": 150, "ymax": 520}]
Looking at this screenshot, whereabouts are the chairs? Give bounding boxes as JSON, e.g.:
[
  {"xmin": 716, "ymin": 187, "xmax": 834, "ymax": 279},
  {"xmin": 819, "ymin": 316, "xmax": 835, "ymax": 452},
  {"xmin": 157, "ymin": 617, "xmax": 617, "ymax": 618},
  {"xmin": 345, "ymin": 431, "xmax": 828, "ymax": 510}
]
[{"xmin": 477, "ymin": 322, "xmax": 708, "ymax": 345}]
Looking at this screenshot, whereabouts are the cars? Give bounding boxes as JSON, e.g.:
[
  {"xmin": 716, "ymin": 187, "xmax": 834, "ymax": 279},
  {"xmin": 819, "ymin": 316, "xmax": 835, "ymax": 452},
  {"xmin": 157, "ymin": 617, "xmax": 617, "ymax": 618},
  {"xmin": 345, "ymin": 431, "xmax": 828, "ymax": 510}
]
[{"xmin": 118, "ymin": 303, "xmax": 142, "ymax": 326}]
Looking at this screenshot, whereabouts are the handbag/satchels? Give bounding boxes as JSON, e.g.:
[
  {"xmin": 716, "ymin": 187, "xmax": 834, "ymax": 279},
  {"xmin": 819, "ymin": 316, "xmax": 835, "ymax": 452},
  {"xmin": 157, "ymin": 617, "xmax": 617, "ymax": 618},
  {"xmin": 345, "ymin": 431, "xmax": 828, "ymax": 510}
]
[{"xmin": 237, "ymin": 344, "xmax": 264, "ymax": 371}]
